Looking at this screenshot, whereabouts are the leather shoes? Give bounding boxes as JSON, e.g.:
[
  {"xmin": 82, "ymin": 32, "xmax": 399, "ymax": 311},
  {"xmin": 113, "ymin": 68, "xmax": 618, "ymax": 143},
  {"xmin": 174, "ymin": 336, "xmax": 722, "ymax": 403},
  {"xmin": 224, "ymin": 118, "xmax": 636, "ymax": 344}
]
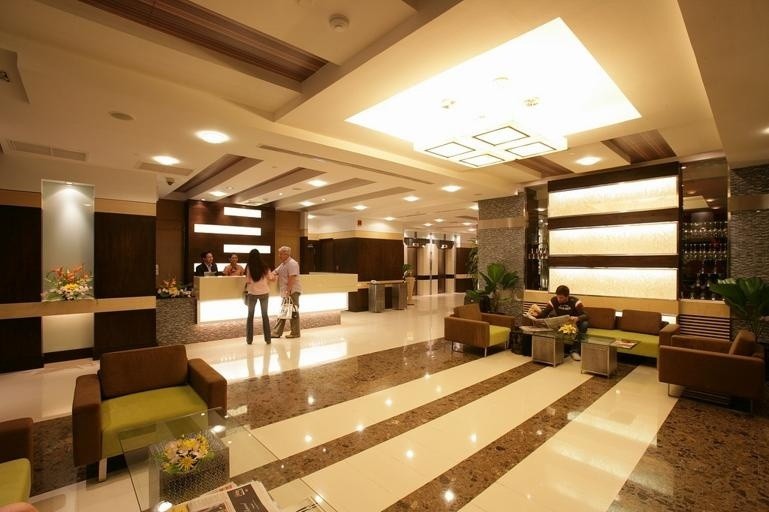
[
  {"xmin": 286, "ymin": 333, "xmax": 299, "ymax": 338},
  {"xmin": 270, "ymin": 332, "xmax": 279, "ymax": 338}
]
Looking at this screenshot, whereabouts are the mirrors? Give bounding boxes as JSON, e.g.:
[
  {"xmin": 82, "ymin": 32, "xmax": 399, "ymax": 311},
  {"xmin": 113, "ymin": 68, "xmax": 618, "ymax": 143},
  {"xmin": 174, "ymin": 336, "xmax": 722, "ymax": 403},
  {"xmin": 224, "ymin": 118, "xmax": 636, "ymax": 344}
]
[
  {"xmin": 682, "ymin": 157, "xmax": 731, "ymax": 301},
  {"xmin": 525, "ymin": 183, "xmax": 549, "ymax": 292}
]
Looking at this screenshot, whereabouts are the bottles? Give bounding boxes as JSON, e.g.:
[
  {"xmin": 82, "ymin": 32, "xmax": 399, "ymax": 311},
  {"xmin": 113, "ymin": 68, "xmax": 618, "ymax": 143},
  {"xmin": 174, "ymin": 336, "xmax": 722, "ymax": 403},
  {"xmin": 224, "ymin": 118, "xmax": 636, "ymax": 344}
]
[
  {"xmin": 682, "ymin": 221, "xmax": 728, "ymax": 239},
  {"xmin": 681, "ymin": 242, "xmax": 728, "ymax": 261},
  {"xmin": 527, "ymin": 246, "xmax": 548, "ymax": 259},
  {"xmin": 678, "ymin": 264, "xmax": 730, "ymax": 301}
]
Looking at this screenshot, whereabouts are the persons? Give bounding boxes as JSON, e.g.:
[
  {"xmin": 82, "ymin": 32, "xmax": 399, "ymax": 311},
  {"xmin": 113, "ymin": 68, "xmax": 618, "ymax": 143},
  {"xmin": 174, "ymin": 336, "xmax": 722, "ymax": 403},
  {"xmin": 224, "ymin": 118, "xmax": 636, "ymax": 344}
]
[
  {"xmin": 224, "ymin": 254, "xmax": 244, "ymax": 275},
  {"xmin": 531, "ymin": 285, "xmax": 588, "ymax": 362},
  {"xmin": 271, "ymin": 246, "xmax": 301, "ymax": 338},
  {"xmin": 196, "ymin": 250, "xmax": 218, "ymax": 276},
  {"xmin": 245, "ymin": 249, "xmax": 275, "ymax": 344}
]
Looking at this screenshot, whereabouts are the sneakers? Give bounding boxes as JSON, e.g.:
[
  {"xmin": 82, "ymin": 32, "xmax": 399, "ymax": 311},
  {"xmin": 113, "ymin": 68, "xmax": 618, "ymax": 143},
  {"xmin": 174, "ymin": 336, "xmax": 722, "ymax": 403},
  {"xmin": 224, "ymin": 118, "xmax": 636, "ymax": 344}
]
[{"xmin": 571, "ymin": 351, "xmax": 581, "ymax": 360}]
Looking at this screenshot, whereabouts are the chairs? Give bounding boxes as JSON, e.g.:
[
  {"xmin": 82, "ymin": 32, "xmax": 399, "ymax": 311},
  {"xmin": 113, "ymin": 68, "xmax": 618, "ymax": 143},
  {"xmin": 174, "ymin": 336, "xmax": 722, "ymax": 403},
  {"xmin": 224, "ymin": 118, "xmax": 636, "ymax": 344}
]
[
  {"xmin": 71, "ymin": 344, "xmax": 227, "ymax": 481},
  {"xmin": 656, "ymin": 329, "xmax": 765, "ymax": 417},
  {"xmin": 0, "ymin": 417, "xmax": 34, "ymax": 512},
  {"xmin": 444, "ymin": 303, "xmax": 516, "ymax": 358}
]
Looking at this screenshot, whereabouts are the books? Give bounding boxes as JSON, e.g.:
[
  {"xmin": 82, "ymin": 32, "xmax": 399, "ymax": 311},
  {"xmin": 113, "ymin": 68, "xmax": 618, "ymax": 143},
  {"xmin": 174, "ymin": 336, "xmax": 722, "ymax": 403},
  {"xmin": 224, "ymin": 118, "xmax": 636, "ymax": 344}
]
[{"xmin": 610, "ymin": 339, "xmax": 638, "ymax": 350}]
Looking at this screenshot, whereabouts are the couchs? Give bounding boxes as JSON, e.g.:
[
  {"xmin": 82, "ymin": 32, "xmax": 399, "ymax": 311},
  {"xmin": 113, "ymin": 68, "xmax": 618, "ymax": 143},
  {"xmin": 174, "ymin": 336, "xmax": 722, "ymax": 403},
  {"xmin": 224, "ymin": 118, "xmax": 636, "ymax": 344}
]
[{"xmin": 524, "ymin": 304, "xmax": 680, "ymax": 361}]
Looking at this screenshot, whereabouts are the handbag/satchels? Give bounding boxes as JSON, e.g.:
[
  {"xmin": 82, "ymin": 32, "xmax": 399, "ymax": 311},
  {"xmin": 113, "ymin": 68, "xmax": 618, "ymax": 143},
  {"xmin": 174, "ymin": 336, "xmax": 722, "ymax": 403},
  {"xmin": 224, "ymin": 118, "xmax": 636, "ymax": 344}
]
[
  {"xmin": 241, "ymin": 283, "xmax": 249, "ymax": 305},
  {"xmin": 277, "ymin": 296, "xmax": 299, "ymax": 320}
]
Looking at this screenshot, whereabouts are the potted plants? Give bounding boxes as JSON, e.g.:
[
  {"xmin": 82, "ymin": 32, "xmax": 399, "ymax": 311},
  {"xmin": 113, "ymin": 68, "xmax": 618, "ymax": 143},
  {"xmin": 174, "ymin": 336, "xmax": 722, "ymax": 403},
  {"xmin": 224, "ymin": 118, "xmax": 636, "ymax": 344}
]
[
  {"xmin": 402, "ymin": 263, "xmax": 415, "ymax": 278},
  {"xmin": 477, "ymin": 261, "xmax": 522, "ymax": 316}
]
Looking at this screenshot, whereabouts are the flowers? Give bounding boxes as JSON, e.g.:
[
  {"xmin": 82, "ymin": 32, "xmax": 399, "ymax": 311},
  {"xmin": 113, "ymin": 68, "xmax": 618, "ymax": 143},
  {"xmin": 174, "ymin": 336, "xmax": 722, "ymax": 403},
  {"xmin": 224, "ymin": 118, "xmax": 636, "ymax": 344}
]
[
  {"xmin": 154, "ymin": 270, "xmax": 195, "ymax": 297},
  {"xmin": 149, "ymin": 432, "xmax": 216, "ymax": 475},
  {"xmin": 40, "ymin": 262, "xmax": 97, "ymax": 300},
  {"xmin": 557, "ymin": 323, "xmax": 577, "ymax": 336}
]
